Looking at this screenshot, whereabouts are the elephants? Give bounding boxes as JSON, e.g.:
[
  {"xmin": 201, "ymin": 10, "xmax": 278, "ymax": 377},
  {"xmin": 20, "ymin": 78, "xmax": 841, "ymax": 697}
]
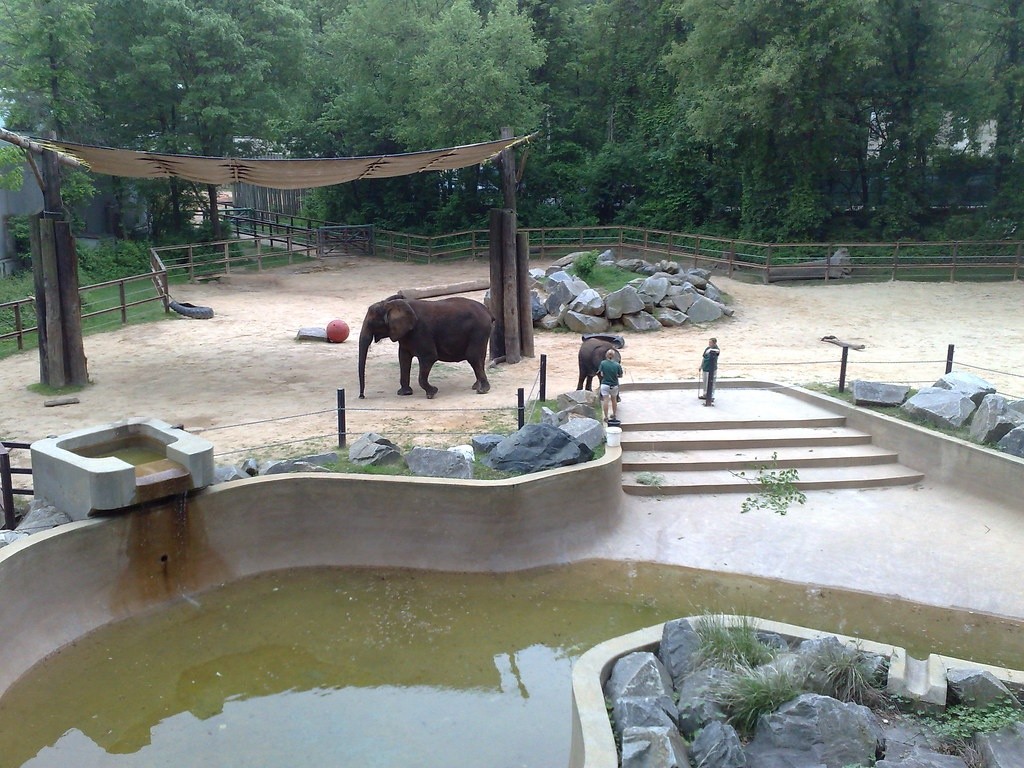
[
  {"xmin": 357, "ymin": 294, "xmax": 496, "ymax": 400},
  {"xmin": 577, "ymin": 338, "xmax": 622, "ymax": 402}
]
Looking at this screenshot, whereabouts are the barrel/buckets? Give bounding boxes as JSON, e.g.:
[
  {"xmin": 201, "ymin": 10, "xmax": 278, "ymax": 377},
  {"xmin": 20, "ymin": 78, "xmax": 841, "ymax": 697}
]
[
  {"xmin": 605, "ymin": 426, "xmax": 622, "ymax": 447},
  {"xmin": 606, "ymin": 419, "xmax": 621, "ymax": 427}
]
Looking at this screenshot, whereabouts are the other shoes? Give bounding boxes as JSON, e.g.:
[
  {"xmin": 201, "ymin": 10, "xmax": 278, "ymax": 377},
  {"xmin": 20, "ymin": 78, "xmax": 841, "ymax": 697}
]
[
  {"xmin": 610, "ymin": 415, "xmax": 616, "ymax": 418},
  {"xmin": 604, "ymin": 418, "xmax": 608, "ymax": 422},
  {"xmin": 699, "ymin": 395, "xmax": 706, "ymax": 399}
]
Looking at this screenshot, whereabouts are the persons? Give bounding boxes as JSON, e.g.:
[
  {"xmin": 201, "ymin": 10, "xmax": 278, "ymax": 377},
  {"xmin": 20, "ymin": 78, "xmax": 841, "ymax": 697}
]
[
  {"xmin": 698, "ymin": 338, "xmax": 720, "ymax": 401},
  {"xmin": 598, "ymin": 349, "xmax": 623, "ymax": 421}
]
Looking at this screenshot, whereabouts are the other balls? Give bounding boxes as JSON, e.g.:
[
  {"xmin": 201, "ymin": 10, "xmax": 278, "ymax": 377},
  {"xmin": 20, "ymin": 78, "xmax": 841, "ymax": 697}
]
[{"xmin": 326, "ymin": 319, "xmax": 350, "ymax": 343}]
